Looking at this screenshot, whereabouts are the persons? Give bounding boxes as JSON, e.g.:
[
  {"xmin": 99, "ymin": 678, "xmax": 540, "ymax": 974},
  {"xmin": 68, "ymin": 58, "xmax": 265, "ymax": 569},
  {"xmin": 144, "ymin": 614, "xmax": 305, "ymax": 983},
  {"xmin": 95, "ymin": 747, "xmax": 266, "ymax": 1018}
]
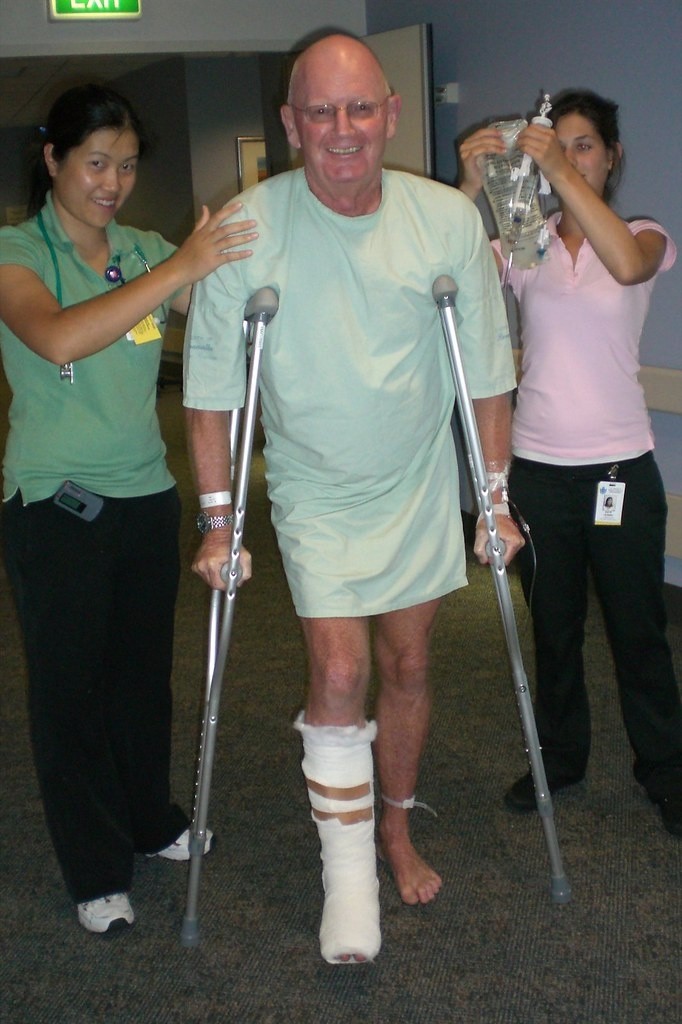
[
  {"xmin": 182, "ymin": 34, "xmax": 525, "ymax": 964},
  {"xmin": 603, "ymin": 497, "xmax": 614, "ymax": 511},
  {"xmin": 0, "ymin": 83, "xmax": 260, "ymax": 932},
  {"xmin": 457, "ymin": 93, "xmax": 682, "ymax": 812}
]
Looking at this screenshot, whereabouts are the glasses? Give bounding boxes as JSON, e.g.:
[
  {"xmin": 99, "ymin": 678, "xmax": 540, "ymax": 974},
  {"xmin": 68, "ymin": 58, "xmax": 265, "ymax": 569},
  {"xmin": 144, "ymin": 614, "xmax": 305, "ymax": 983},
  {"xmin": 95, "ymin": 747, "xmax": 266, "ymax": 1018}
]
[{"xmin": 289, "ymin": 93, "xmax": 392, "ymax": 123}]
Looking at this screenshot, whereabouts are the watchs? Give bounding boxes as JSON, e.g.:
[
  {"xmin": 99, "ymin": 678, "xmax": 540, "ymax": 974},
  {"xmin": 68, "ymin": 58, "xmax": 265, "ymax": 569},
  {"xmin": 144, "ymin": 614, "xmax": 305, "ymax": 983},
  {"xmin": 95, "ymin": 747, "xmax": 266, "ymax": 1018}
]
[{"xmin": 197, "ymin": 512, "xmax": 233, "ymax": 533}]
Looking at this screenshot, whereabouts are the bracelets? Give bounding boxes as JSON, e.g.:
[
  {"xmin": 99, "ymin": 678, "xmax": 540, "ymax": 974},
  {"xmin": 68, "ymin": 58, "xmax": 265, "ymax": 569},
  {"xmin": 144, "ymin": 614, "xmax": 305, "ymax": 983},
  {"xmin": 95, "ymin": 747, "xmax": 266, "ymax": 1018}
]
[{"xmin": 199, "ymin": 491, "xmax": 231, "ymax": 509}]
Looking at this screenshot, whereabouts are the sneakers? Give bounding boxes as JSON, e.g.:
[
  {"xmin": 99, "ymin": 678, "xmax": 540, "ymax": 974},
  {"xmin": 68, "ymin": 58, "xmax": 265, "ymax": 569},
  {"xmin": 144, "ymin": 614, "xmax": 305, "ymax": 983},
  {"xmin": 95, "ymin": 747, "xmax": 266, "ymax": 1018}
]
[
  {"xmin": 77, "ymin": 892, "xmax": 135, "ymax": 934},
  {"xmin": 647, "ymin": 785, "xmax": 682, "ymax": 838},
  {"xmin": 504, "ymin": 768, "xmax": 586, "ymax": 812},
  {"xmin": 145, "ymin": 824, "xmax": 216, "ymax": 861}
]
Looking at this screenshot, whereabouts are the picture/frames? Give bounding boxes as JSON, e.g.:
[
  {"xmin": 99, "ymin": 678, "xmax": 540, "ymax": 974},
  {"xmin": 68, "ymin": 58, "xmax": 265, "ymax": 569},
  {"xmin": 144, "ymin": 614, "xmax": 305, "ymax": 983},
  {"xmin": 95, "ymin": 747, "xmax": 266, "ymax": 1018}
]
[{"xmin": 237, "ymin": 136, "xmax": 268, "ymax": 194}]
